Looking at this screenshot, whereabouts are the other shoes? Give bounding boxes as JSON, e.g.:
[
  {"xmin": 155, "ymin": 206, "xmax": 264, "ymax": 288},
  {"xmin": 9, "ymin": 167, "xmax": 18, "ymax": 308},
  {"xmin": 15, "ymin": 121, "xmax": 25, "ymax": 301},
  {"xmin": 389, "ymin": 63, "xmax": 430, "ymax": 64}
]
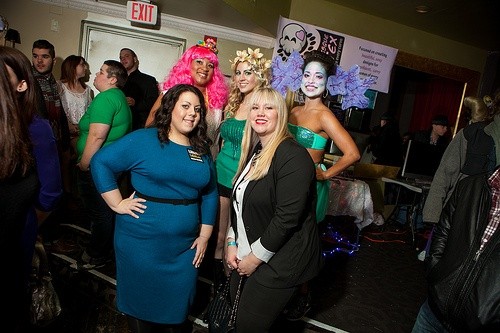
[
  {"xmin": 70, "ymin": 260, "xmax": 105, "ymax": 269},
  {"xmin": 417, "ymin": 250, "xmax": 427, "ymax": 262}
]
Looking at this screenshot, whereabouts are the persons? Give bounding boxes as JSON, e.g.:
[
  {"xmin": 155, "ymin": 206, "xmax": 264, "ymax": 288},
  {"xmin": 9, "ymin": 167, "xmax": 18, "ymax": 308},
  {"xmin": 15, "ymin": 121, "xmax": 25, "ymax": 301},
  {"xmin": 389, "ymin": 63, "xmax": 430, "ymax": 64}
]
[
  {"xmin": 197, "ymin": 88, "xmax": 324, "ymax": 333},
  {"xmin": 215, "ymin": 46, "xmax": 272, "ymax": 295},
  {"xmin": 0, "ymin": 46, "xmax": 62, "ymax": 274},
  {"xmin": 424, "ymin": 95, "xmax": 500, "ymax": 223},
  {"xmin": 368, "ymin": 111, "xmax": 402, "ymax": 167},
  {"xmin": 145, "ymin": 40, "xmax": 229, "ymax": 162},
  {"xmin": 285, "ymin": 52, "xmax": 360, "ymax": 320},
  {"xmin": 55, "ymin": 54, "xmax": 93, "ymax": 150},
  {"xmin": 31, "ymin": 39, "xmax": 65, "ymax": 157},
  {"xmin": 406, "ymin": 165, "xmax": 500, "ymax": 333},
  {"xmin": 89, "ymin": 83, "xmax": 219, "ymax": 333},
  {"xmin": 70, "ymin": 60, "xmax": 132, "ymax": 273},
  {"xmin": 0, "ymin": 56, "xmax": 37, "ymax": 333},
  {"xmin": 119, "ymin": 48, "xmax": 158, "ymax": 133},
  {"xmin": 412, "ymin": 113, "xmax": 450, "ymax": 176}
]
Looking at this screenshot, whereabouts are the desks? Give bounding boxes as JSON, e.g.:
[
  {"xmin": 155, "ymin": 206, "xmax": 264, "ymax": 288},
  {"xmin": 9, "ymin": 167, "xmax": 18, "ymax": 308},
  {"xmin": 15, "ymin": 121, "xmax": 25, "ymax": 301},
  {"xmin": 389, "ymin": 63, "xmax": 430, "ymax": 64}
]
[{"xmin": 381, "ymin": 175, "xmax": 432, "ymax": 249}]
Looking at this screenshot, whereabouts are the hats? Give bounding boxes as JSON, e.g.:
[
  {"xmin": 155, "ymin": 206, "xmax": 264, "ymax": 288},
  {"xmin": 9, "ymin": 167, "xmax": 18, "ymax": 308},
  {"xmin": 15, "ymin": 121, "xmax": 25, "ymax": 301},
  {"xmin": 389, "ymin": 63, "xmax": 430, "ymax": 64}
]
[{"xmin": 430, "ymin": 114, "xmax": 448, "ymax": 126}]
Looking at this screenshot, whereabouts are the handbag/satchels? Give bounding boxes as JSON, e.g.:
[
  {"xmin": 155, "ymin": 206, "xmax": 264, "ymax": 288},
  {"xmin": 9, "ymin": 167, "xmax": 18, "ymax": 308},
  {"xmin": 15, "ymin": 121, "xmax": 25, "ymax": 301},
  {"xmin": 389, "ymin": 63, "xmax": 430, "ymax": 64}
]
[
  {"xmin": 203, "ymin": 268, "xmax": 244, "ymax": 333},
  {"xmin": 30, "ymin": 273, "xmax": 61, "ymax": 322}
]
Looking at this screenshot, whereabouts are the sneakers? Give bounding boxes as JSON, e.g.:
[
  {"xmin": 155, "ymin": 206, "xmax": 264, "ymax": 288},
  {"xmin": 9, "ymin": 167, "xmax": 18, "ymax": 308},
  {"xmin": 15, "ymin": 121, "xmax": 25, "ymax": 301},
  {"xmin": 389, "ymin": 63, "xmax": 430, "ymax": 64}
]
[{"xmin": 287, "ymin": 295, "xmax": 312, "ymax": 322}]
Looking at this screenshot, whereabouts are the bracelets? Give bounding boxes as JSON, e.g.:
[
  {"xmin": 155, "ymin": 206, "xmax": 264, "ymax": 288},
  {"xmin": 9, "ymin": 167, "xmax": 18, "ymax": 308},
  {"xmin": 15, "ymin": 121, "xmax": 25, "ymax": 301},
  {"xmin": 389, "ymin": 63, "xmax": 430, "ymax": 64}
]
[{"xmin": 226, "ymin": 242, "xmax": 238, "ymax": 248}]
